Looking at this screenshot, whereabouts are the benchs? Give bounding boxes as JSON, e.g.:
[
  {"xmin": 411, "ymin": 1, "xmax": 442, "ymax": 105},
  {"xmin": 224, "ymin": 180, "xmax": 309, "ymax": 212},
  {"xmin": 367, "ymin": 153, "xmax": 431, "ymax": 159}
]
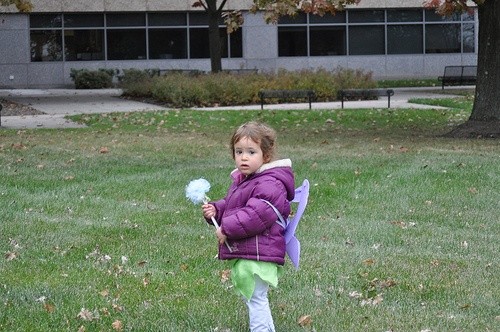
[
  {"xmin": 336, "ymin": 88, "xmax": 394, "ymax": 108},
  {"xmin": 258, "ymin": 89, "xmax": 315, "ymax": 110},
  {"xmin": 439, "ymin": 65, "xmax": 478, "ymax": 89}
]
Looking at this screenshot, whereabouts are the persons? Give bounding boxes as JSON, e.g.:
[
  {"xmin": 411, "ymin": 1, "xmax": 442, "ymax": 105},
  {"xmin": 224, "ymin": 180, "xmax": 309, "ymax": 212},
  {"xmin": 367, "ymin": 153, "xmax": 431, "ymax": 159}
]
[{"xmin": 201, "ymin": 120, "xmax": 295, "ymax": 332}]
